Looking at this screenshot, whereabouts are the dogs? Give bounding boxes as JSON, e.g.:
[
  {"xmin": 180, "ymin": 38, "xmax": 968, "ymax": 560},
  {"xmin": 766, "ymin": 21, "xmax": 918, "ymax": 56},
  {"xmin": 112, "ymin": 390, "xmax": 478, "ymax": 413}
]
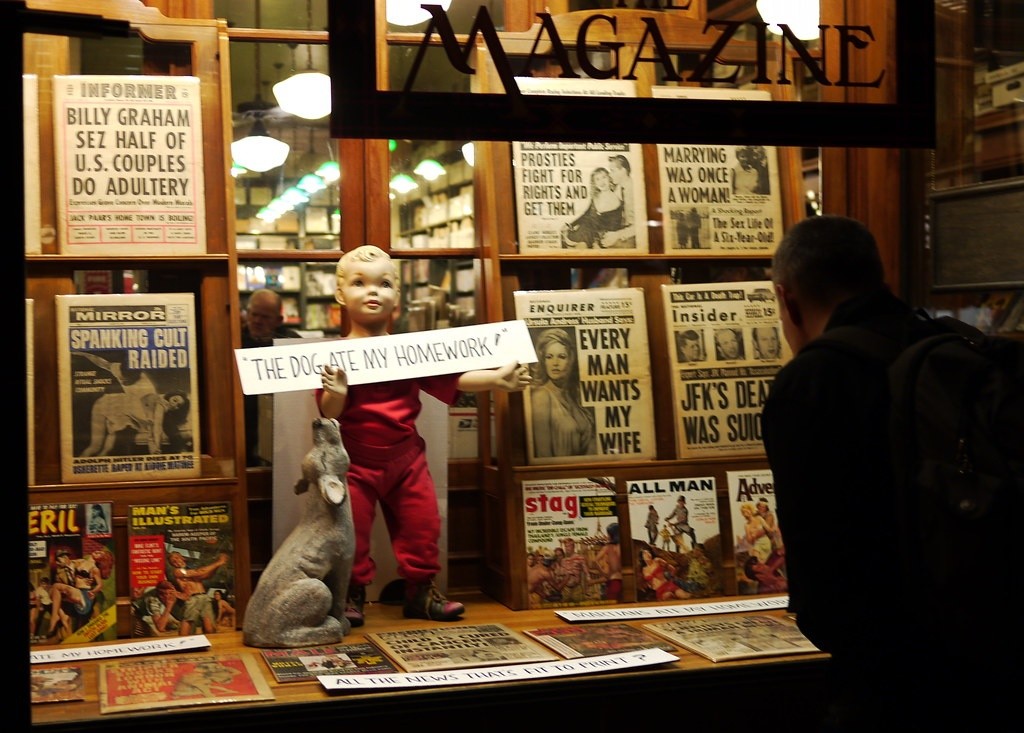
[{"xmin": 241, "ymin": 415, "xmax": 354, "ymax": 648}]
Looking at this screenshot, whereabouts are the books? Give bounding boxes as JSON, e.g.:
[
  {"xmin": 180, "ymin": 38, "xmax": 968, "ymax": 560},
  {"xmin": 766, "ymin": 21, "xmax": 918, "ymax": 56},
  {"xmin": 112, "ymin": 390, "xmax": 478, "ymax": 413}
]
[
  {"xmin": 236, "ymin": 140, "xmax": 478, "ymax": 340},
  {"xmin": 654, "ymin": 89, "xmax": 782, "ymax": 258},
  {"xmin": 54, "ymin": 293, "xmax": 202, "ymax": 483},
  {"xmin": 127, "ymin": 501, "xmax": 236, "ymax": 638},
  {"xmin": 52, "ymin": 74, "xmax": 209, "ymax": 254},
  {"xmin": 31, "ymin": 612, "xmax": 822, "ymax": 716},
  {"xmin": 28, "ymin": 500, "xmax": 118, "ymax": 645},
  {"xmin": 512, "ymin": 78, "xmax": 650, "ymax": 258},
  {"xmin": 514, "ymin": 281, "xmax": 796, "ymax": 610}
]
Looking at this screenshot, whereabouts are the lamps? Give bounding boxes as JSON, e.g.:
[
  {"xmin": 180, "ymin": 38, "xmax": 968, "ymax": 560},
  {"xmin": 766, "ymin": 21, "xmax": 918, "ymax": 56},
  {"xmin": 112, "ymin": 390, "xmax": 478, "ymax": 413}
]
[{"xmin": 231, "ymin": 0, "xmax": 475, "ymax": 223}]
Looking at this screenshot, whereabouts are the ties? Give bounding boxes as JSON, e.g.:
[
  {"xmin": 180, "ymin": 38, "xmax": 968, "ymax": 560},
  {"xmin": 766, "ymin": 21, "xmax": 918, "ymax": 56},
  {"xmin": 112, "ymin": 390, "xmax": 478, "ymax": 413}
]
[{"xmin": 621, "ymin": 187, "xmax": 625, "ymax": 212}]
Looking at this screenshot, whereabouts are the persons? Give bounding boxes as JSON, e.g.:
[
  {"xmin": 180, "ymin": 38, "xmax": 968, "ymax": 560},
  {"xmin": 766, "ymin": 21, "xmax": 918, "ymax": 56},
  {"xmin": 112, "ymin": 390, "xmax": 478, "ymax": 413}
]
[
  {"xmin": 72, "ymin": 351, "xmax": 190, "ymax": 457},
  {"xmin": 529, "ymin": 329, "xmax": 597, "ymax": 458},
  {"xmin": 560, "ymin": 155, "xmax": 638, "ymax": 250},
  {"xmin": 678, "ymin": 330, "xmax": 702, "ymax": 363},
  {"xmin": 240, "ymin": 290, "xmax": 302, "ymax": 468},
  {"xmin": 314, "ymin": 246, "xmax": 532, "ymax": 626},
  {"xmin": 730, "ymin": 149, "xmax": 759, "ymax": 195},
  {"xmin": 753, "ymin": 327, "xmax": 781, "ymax": 359},
  {"xmin": 760, "ymin": 215, "xmax": 1024, "ymax": 733},
  {"xmin": 676, "ymin": 208, "xmax": 702, "ymax": 249},
  {"xmin": 715, "ymin": 329, "xmax": 744, "ymax": 362}
]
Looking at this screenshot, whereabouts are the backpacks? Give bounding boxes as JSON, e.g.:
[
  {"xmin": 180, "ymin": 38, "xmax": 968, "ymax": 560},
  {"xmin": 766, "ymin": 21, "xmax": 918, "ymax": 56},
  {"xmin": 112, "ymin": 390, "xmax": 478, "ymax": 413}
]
[{"xmin": 796, "ymin": 306, "xmax": 1024, "ymax": 659}]
